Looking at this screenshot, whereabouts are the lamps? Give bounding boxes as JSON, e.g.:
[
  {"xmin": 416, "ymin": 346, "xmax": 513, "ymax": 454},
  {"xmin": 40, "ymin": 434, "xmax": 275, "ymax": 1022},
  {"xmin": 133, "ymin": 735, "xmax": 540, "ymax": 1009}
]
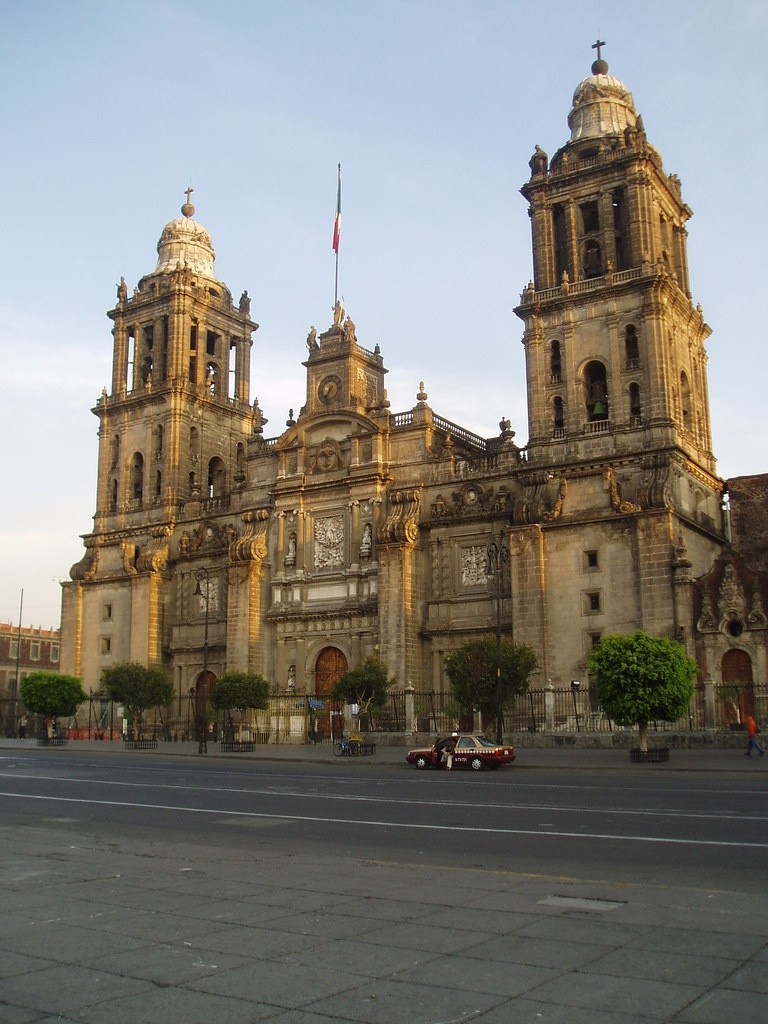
[
  {"xmin": 571, "ymin": 679, "xmax": 580, "ymax": 688},
  {"xmin": 373, "ymin": 643, "xmax": 380, "ymax": 652}
]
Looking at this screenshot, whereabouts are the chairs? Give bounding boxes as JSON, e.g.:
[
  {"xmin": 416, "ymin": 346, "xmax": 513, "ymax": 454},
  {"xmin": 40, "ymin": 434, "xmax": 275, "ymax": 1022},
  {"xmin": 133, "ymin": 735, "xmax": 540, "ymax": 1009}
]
[{"xmin": 561, "ymin": 711, "xmax": 604, "ymax": 733}]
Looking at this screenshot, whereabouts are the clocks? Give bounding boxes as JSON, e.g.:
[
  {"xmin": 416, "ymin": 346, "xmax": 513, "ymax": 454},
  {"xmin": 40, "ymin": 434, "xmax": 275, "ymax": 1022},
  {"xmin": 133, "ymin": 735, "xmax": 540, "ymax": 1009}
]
[{"xmin": 315, "ymin": 372, "xmax": 344, "ymax": 407}]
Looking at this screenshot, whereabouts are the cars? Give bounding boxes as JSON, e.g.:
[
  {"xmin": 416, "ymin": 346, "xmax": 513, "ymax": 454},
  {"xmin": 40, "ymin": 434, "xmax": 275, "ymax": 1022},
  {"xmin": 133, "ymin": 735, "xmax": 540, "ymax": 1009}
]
[{"xmin": 406, "ymin": 732, "xmax": 516, "ymax": 771}]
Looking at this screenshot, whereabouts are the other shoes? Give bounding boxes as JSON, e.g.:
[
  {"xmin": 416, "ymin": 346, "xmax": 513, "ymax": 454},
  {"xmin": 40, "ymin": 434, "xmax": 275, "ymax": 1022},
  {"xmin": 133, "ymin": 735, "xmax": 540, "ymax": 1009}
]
[{"xmin": 447, "ymin": 768, "xmax": 451, "ymax": 771}]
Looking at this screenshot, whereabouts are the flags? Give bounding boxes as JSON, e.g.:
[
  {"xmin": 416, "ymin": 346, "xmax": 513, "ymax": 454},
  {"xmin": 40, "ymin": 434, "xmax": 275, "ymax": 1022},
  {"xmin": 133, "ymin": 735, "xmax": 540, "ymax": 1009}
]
[{"xmin": 332, "ymin": 163, "xmax": 342, "ymax": 253}]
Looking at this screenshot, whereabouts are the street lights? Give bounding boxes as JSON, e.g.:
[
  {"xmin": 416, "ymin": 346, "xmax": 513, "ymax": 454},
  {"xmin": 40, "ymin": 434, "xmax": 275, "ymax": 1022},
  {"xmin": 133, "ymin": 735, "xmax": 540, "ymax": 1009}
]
[
  {"xmin": 481, "ymin": 542, "xmax": 510, "ymax": 748},
  {"xmin": 191, "ymin": 567, "xmax": 210, "ymax": 755}
]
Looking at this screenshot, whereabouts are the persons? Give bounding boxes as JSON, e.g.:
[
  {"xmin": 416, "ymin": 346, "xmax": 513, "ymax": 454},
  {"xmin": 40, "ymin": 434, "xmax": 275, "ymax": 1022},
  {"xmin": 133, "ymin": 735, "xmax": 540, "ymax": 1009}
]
[
  {"xmin": 441, "ymin": 740, "xmax": 456, "ymax": 771},
  {"xmin": 17, "ymin": 715, "xmax": 27, "ymax": 741},
  {"xmin": 742, "ymin": 712, "xmax": 765, "ymax": 756}
]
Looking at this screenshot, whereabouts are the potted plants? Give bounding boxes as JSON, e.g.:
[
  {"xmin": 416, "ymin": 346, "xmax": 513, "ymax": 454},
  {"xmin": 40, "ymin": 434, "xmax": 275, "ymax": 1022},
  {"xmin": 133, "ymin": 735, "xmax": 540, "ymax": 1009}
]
[
  {"xmin": 308, "ymin": 709, "xmax": 328, "ymax": 742},
  {"xmin": 251, "ymin": 702, "xmax": 276, "ymax": 744},
  {"xmin": 401, "ymin": 695, "xmax": 430, "ymax": 732},
  {"xmin": 443, "ymin": 699, "xmax": 474, "ymax": 732},
  {"xmin": 714, "ymin": 678, "xmax": 747, "ymax": 731}
]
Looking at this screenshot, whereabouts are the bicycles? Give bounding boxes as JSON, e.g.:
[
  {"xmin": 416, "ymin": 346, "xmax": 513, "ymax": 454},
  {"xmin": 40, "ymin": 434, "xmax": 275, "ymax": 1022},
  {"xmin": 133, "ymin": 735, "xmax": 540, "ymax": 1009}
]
[{"xmin": 333, "ymin": 735, "xmax": 361, "ymax": 757}]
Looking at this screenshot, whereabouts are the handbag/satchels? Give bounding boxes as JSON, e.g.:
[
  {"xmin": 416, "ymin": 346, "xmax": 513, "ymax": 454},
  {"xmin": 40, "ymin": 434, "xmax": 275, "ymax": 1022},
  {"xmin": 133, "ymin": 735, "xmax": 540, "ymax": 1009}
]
[{"xmin": 441, "ymin": 754, "xmax": 448, "ymax": 762}]
